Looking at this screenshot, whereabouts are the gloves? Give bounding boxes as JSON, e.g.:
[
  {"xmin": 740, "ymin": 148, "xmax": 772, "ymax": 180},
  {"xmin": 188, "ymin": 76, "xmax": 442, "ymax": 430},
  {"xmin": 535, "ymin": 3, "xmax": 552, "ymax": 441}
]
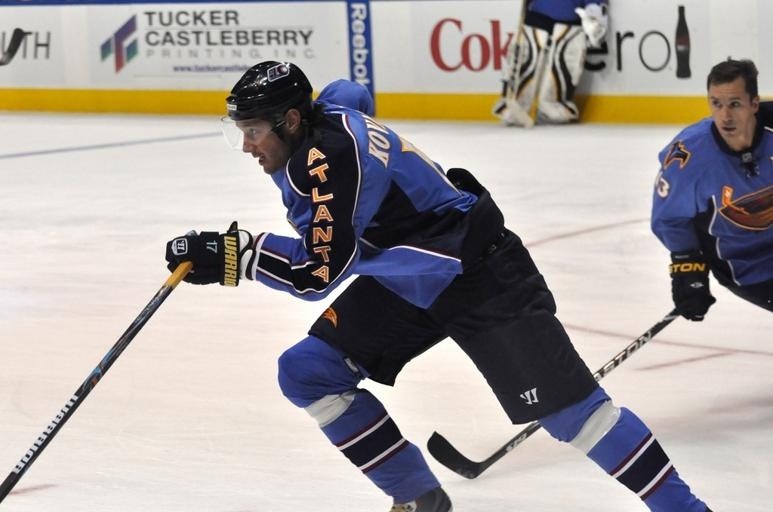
[
  {"xmin": 668, "ymin": 248, "xmax": 717, "ymax": 324},
  {"xmin": 166, "ymin": 220, "xmax": 254, "ymax": 289}
]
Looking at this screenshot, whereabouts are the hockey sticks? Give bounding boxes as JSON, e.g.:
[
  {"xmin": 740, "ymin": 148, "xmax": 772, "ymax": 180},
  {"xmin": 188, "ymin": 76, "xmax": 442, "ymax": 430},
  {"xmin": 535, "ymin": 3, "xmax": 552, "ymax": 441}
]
[
  {"xmin": 503, "ymin": 1, "xmax": 536, "ymax": 128},
  {"xmin": 426, "ymin": 307, "xmax": 682, "ymax": 479}
]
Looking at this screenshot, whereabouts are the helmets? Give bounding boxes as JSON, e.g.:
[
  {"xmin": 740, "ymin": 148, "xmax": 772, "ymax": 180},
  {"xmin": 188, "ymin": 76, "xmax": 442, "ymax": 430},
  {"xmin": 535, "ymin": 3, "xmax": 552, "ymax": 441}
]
[{"xmin": 223, "ymin": 58, "xmax": 313, "ymax": 121}]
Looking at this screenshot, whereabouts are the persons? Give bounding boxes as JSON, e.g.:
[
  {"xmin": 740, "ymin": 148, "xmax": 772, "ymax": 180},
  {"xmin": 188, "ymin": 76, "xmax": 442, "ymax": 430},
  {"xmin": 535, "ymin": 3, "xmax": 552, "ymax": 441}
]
[
  {"xmin": 166, "ymin": 59, "xmax": 711, "ymax": 512},
  {"xmin": 651, "ymin": 59, "xmax": 773, "ymax": 321},
  {"xmin": 497, "ymin": 1, "xmax": 609, "ymax": 127}
]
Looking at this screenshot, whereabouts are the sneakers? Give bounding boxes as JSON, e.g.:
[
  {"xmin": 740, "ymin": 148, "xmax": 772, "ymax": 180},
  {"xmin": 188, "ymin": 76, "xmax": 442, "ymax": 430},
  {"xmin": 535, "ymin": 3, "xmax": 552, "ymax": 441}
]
[{"xmin": 388, "ymin": 482, "xmax": 452, "ymax": 512}]
